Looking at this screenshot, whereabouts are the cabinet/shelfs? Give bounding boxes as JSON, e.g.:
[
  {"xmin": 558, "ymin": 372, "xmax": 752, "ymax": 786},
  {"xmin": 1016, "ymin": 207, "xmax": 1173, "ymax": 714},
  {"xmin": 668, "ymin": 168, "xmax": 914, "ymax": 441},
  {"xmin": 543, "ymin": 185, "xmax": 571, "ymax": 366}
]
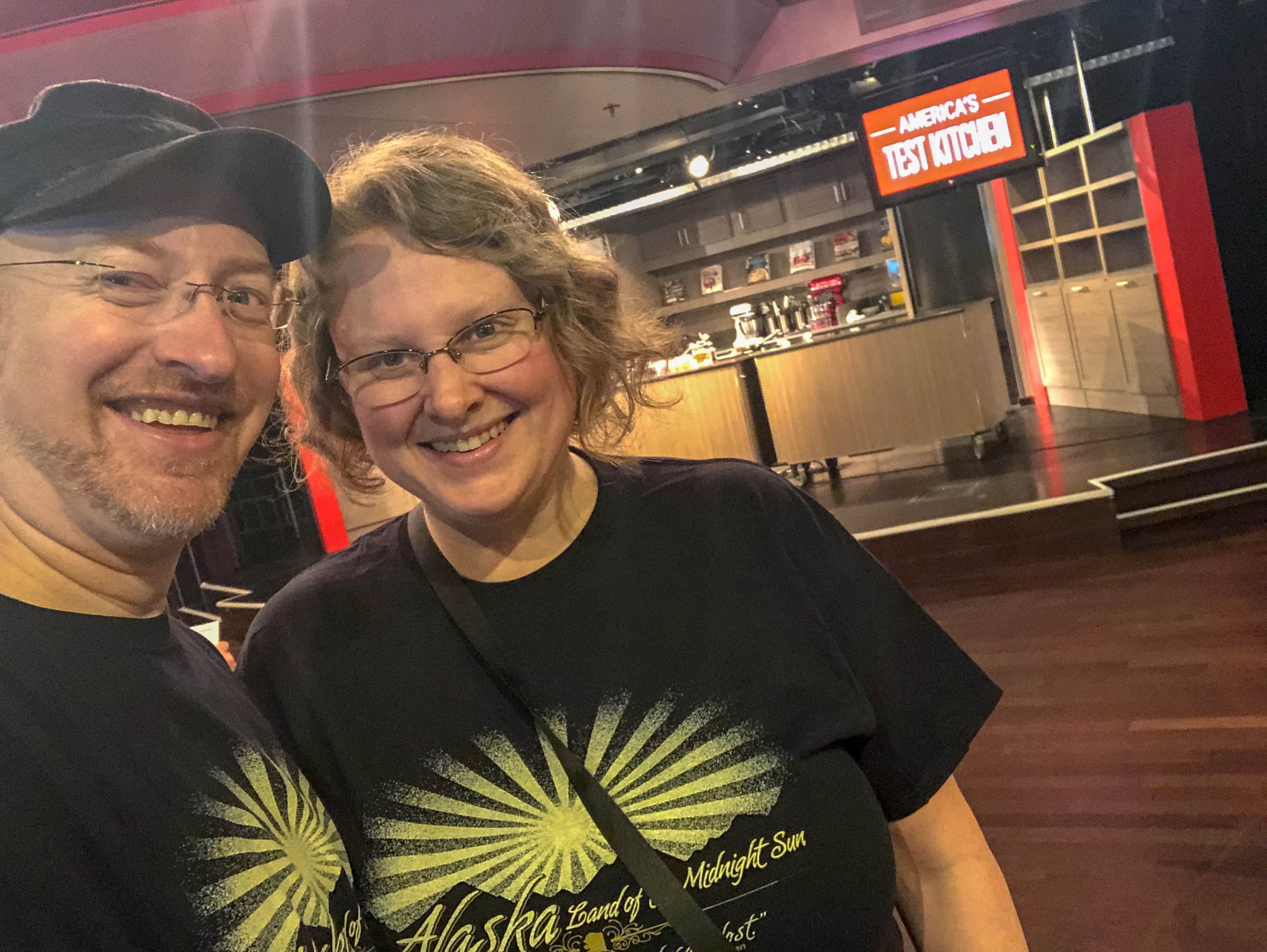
[
  {"xmin": 724, "ymin": 175, "xmax": 784, "ymax": 236},
  {"xmin": 772, "ymin": 148, "xmax": 872, "ymax": 222},
  {"xmin": 999, "ymin": 102, "xmax": 1249, "ymax": 421},
  {"xmin": 637, "ymin": 192, "xmax": 732, "ymax": 260}
]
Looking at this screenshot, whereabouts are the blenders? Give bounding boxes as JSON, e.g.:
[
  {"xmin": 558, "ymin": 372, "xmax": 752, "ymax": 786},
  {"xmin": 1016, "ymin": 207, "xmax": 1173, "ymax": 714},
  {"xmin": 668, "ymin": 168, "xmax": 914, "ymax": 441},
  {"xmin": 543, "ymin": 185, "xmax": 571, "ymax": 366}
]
[
  {"xmin": 729, "ymin": 303, "xmax": 764, "ymax": 346},
  {"xmin": 810, "ymin": 275, "xmax": 845, "ymax": 330}
]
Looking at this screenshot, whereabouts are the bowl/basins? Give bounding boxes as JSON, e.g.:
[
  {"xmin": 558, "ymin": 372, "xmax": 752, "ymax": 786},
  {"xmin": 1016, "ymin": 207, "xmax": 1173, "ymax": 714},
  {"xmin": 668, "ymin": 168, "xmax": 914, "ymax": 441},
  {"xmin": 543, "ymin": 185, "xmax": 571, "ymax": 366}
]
[
  {"xmin": 808, "ymin": 300, "xmax": 833, "ymax": 324},
  {"xmin": 861, "ymin": 305, "xmax": 881, "ymax": 317},
  {"xmin": 738, "ymin": 314, "xmax": 763, "ymax": 340}
]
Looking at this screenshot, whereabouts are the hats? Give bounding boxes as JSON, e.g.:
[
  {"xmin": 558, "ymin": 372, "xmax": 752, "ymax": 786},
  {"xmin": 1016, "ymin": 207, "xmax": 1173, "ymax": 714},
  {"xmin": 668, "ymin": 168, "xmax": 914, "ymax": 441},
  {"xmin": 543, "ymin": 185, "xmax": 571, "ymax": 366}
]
[{"xmin": 3, "ymin": 80, "xmax": 333, "ymax": 266}]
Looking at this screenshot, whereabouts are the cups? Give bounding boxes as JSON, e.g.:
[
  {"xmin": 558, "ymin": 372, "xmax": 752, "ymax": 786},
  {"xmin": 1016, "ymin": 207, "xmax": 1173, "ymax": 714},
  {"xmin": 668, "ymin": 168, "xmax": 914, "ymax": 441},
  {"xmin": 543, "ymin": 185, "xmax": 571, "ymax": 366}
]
[{"xmin": 765, "ymin": 312, "xmax": 805, "ymax": 335}]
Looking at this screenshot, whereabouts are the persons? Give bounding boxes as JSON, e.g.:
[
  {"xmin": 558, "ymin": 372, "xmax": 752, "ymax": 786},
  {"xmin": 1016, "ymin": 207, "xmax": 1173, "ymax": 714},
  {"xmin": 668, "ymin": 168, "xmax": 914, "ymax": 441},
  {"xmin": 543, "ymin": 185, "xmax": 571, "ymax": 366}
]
[
  {"xmin": 242, "ymin": 130, "xmax": 1031, "ymax": 952},
  {"xmin": 1, "ymin": 80, "xmax": 374, "ymax": 952}
]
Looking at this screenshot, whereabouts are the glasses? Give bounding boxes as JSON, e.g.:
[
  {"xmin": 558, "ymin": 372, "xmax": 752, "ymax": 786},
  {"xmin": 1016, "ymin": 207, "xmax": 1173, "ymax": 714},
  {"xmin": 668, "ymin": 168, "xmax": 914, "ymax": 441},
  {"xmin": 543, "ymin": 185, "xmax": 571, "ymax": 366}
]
[
  {"xmin": 0, "ymin": 255, "xmax": 305, "ymax": 331},
  {"xmin": 324, "ymin": 298, "xmax": 556, "ymax": 411}
]
